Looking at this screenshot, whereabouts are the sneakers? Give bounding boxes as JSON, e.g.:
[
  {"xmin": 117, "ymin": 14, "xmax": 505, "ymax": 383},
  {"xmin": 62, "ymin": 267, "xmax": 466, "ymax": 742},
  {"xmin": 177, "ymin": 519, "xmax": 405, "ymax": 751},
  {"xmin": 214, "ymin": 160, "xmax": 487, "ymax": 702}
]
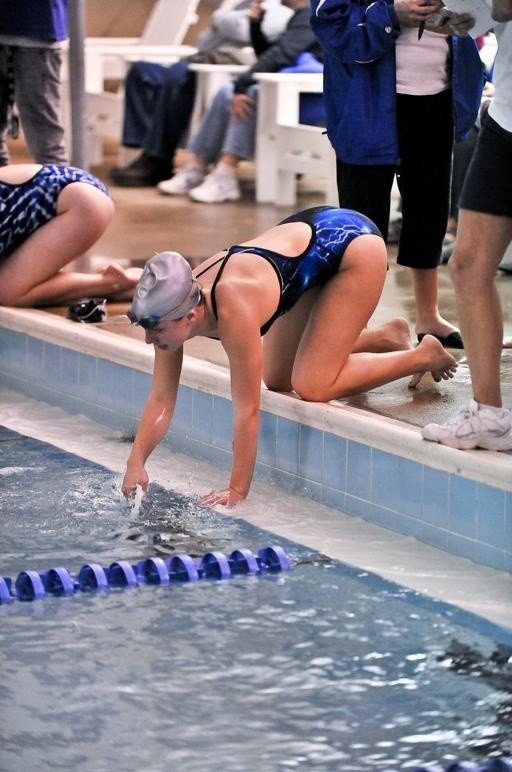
[
  {"xmin": 418, "ymin": 329, "xmax": 464, "ymax": 349},
  {"xmin": 190, "ymin": 174, "xmax": 239, "ymax": 202},
  {"xmin": 69, "ymin": 299, "xmax": 109, "ymax": 322},
  {"xmin": 111, "ymin": 154, "xmax": 173, "ymax": 185},
  {"xmin": 422, "ymin": 398, "xmax": 512, "ymax": 451},
  {"xmin": 159, "ymin": 167, "xmax": 205, "ymax": 194}
]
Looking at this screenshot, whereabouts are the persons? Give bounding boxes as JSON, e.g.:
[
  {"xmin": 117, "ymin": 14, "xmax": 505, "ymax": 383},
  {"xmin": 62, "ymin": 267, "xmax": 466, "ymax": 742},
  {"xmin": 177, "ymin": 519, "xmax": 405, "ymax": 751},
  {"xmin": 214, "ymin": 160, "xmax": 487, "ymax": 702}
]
[
  {"xmin": 420, "ymin": 0, "xmax": 512, "ymax": 453},
  {"xmin": 0, "ymin": 161, "xmax": 146, "ymax": 308},
  {"xmin": 121, "ymin": 206, "xmax": 458, "ymax": 511},
  {"xmin": 108, "ymin": 0, "xmax": 295, "ymax": 186},
  {"xmin": 385, "ymin": 59, "xmax": 495, "ymax": 244},
  {"xmin": 2, "ymin": 1, "xmax": 71, "ymax": 167},
  {"xmin": 158, "ymin": 0, "xmax": 326, "ymax": 203},
  {"xmin": 438, "ymin": 239, "xmax": 511, "ymax": 276},
  {"xmin": 309, "ymin": 1, "xmax": 484, "ymax": 348}
]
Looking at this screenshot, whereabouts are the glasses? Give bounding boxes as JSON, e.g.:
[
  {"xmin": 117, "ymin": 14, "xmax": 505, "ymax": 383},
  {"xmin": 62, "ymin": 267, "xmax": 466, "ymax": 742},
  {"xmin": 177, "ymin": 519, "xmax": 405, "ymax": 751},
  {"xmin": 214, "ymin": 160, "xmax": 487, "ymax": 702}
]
[{"xmin": 127, "ymin": 274, "xmax": 198, "ymax": 328}]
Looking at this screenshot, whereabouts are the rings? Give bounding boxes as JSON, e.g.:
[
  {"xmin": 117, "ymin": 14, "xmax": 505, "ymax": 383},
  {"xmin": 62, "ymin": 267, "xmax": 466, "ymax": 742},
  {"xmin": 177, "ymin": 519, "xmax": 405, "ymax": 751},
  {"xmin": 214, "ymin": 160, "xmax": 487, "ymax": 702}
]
[
  {"xmin": 454, "ymin": 31, "xmax": 461, "ymax": 36},
  {"xmin": 450, "ymin": 25, "xmax": 455, "ymax": 31}
]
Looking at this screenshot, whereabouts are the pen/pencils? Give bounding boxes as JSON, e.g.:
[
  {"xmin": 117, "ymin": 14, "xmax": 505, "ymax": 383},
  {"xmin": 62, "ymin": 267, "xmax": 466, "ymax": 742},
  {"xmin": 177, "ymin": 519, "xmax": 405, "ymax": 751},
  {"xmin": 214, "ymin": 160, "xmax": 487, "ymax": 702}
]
[{"xmin": 419, "ymin": 0, "xmax": 431, "ymax": 41}]
